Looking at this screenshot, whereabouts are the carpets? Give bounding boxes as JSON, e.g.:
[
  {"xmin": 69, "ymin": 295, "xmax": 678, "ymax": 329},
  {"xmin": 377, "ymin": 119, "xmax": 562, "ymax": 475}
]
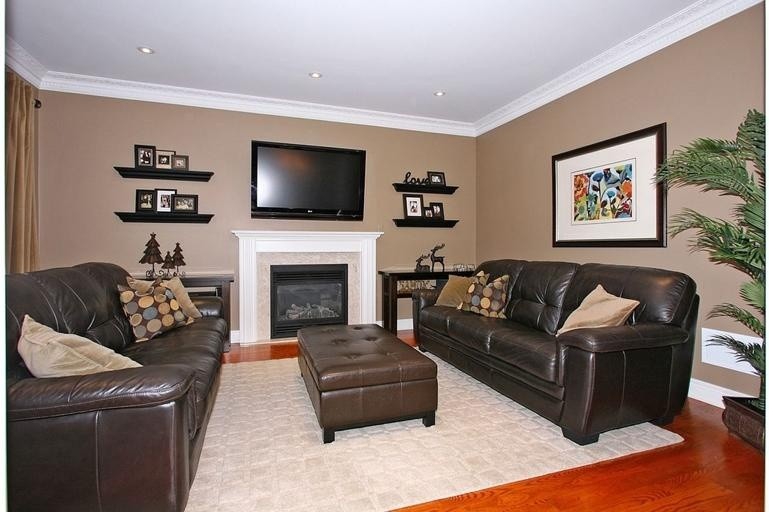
[{"xmin": 184, "ymin": 345, "xmax": 685, "ymax": 512}]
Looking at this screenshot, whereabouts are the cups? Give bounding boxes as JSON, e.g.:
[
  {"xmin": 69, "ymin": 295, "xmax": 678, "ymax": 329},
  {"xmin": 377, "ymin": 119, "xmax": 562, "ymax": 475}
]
[{"xmin": 453, "ymin": 263, "xmax": 475, "ymax": 271}]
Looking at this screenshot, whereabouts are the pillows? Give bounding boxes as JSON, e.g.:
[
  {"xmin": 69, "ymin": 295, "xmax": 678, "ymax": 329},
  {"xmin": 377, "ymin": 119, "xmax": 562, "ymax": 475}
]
[
  {"xmin": 126, "ymin": 275, "xmax": 203, "ymax": 318},
  {"xmin": 434, "ymin": 271, "xmax": 640, "ymax": 337},
  {"xmin": 114, "ymin": 277, "xmax": 194, "ymax": 343},
  {"xmin": 17, "ymin": 314, "xmax": 143, "ymax": 378}
]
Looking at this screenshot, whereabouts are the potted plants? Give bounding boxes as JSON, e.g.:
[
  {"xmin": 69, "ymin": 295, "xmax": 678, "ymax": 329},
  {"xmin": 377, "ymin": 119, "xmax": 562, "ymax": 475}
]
[{"xmin": 650, "ymin": 109, "xmax": 766, "ymax": 454}]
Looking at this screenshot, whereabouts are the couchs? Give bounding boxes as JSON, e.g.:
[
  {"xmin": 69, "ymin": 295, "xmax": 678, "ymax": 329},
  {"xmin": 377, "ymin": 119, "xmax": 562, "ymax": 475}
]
[
  {"xmin": 5, "ymin": 262, "xmax": 228, "ymax": 512},
  {"xmin": 411, "ymin": 260, "xmax": 700, "ymax": 446}
]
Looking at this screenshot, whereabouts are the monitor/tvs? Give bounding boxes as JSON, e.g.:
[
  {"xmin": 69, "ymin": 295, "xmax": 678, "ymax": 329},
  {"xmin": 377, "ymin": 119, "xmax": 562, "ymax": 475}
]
[{"xmin": 250, "ymin": 140, "xmax": 366, "ymax": 222}]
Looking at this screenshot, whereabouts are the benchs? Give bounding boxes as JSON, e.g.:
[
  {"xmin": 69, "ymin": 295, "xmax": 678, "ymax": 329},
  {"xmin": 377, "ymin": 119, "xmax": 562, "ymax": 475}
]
[{"xmin": 297, "ymin": 324, "xmax": 438, "ymax": 443}]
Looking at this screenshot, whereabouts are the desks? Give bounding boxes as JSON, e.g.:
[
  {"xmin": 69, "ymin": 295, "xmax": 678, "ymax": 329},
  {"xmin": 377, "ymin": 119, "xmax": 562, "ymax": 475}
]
[
  {"xmin": 379, "ymin": 271, "xmax": 474, "ymax": 336},
  {"xmin": 135, "ymin": 274, "xmax": 234, "ymax": 352}
]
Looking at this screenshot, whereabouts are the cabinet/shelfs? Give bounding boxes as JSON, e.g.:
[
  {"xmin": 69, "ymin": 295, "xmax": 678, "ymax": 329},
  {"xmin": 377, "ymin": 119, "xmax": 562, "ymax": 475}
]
[
  {"xmin": 392, "ymin": 184, "xmax": 459, "ymax": 228},
  {"xmin": 113, "ymin": 167, "xmax": 215, "ymax": 224}
]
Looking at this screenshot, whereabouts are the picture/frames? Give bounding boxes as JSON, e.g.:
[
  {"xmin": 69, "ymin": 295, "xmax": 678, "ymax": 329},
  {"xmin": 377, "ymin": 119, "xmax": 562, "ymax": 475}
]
[
  {"xmin": 136, "ymin": 189, "xmax": 198, "ymax": 214},
  {"xmin": 553, "ymin": 122, "xmax": 667, "ymax": 248},
  {"xmin": 135, "ymin": 145, "xmax": 189, "ymax": 171},
  {"xmin": 403, "ymin": 194, "xmax": 444, "ymax": 220},
  {"xmin": 428, "ymin": 172, "xmax": 446, "ymax": 186}
]
